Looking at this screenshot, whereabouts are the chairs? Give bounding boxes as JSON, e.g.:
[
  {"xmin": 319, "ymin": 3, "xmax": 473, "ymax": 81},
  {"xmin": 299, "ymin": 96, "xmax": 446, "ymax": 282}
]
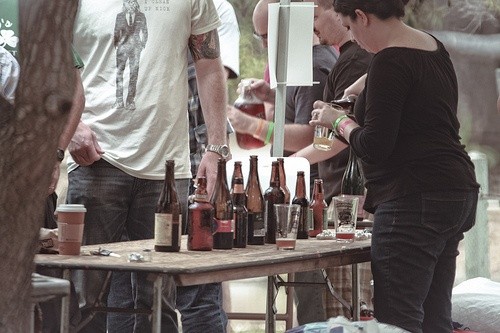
[{"xmin": 30, "ymin": 273, "xmax": 71, "ymax": 333}]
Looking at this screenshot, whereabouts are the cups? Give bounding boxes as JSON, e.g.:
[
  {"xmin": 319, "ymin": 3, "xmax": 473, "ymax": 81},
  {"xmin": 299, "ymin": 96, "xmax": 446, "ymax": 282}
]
[
  {"xmin": 313, "ymin": 103, "xmax": 343, "ymax": 151},
  {"xmin": 331, "ymin": 196, "xmax": 359, "ymax": 244},
  {"xmin": 273, "ymin": 203, "xmax": 301, "ymax": 251},
  {"xmin": 56, "ymin": 204, "xmax": 87, "ymax": 254}
]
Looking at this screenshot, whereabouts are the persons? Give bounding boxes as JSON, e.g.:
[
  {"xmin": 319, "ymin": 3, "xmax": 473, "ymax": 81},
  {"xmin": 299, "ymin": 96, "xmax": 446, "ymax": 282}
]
[
  {"xmin": 0, "ymin": 0, "xmax": 411, "ymax": 333},
  {"xmin": 309, "ymin": 0, "xmax": 481, "ymax": 333}
]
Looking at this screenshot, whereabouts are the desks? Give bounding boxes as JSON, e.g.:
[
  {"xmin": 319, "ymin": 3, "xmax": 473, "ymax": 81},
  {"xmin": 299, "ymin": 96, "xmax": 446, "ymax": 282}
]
[{"xmin": 32, "ymin": 234, "xmax": 371, "ymax": 333}]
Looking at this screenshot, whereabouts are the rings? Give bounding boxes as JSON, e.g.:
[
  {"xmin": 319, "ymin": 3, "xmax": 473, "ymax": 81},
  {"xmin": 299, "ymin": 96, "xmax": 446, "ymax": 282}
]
[
  {"xmin": 50, "ymin": 187, "xmax": 55, "ymax": 190},
  {"xmin": 314, "ymin": 113, "xmax": 317, "ymax": 115}
]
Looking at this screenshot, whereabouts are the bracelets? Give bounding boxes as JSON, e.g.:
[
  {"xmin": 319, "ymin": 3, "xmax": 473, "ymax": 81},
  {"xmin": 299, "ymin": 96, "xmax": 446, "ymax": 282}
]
[
  {"xmin": 254, "ymin": 119, "xmax": 264, "ymax": 138},
  {"xmin": 332, "ymin": 115, "xmax": 347, "ymax": 129},
  {"xmin": 265, "ymin": 122, "xmax": 273, "ymax": 144},
  {"xmin": 340, "ymin": 119, "xmax": 353, "ymax": 136}
]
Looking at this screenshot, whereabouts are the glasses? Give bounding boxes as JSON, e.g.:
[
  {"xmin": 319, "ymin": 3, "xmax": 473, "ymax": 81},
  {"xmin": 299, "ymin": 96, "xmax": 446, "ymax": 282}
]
[{"xmin": 254, "ymin": 32, "xmax": 267, "ymax": 42}]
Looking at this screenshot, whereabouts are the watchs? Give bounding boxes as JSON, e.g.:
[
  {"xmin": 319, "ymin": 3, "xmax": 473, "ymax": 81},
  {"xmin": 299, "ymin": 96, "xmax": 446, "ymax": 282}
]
[
  {"xmin": 206, "ymin": 145, "xmax": 230, "ymax": 159},
  {"xmin": 56, "ymin": 149, "xmax": 64, "ymax": 161}
]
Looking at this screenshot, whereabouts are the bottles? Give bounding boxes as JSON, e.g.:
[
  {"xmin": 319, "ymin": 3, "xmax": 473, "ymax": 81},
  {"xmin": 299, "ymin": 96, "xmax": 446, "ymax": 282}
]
[
  {"xmin": 341, "ymin": 145, "xmax": 366, "ymax": 220},
  {"xmin": 331, "ymin": 94, "xmax": 358, "ymax": 117},
  {"xmin": 153, "ymin": 155, "xmax": 328, "ymax": 253},
  {"xmin": 234, "ymin": 79, "xmax": 269, "ymax": 151}
]
